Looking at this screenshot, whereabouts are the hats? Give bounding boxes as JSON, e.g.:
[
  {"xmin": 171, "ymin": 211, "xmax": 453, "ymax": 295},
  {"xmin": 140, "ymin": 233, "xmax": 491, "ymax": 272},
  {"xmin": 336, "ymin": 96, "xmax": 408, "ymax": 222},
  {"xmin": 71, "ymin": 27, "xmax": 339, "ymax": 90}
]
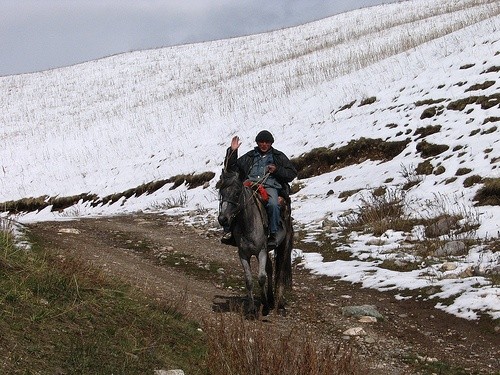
[{"xmin": 255, "ymin": 130, "xmax": 274, "ymax": 143}]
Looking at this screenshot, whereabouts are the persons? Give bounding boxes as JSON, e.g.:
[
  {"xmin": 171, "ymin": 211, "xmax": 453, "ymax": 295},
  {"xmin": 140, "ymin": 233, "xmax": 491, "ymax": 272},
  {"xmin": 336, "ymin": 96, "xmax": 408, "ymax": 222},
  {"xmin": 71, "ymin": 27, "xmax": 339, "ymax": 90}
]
[{"xmin": 221, "ymin": 130, "xmax": 298, "ymax": 247}]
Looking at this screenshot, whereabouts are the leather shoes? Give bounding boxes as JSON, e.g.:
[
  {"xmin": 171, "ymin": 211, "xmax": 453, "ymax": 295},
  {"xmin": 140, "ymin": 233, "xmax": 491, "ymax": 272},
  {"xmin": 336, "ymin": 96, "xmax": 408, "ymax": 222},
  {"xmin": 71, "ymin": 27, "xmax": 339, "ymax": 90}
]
[{"xmin": 267, "ymin": 236, "xmax": 276, "ymax": 246}]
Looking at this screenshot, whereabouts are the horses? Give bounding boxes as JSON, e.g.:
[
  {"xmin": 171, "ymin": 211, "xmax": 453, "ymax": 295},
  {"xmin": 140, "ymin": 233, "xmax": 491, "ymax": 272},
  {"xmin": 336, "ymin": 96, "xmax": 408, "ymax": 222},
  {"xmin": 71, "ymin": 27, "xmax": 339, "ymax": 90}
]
[{"xmin": 215, "ymin": 167, "xmax": 295, "ymax": 316}]
